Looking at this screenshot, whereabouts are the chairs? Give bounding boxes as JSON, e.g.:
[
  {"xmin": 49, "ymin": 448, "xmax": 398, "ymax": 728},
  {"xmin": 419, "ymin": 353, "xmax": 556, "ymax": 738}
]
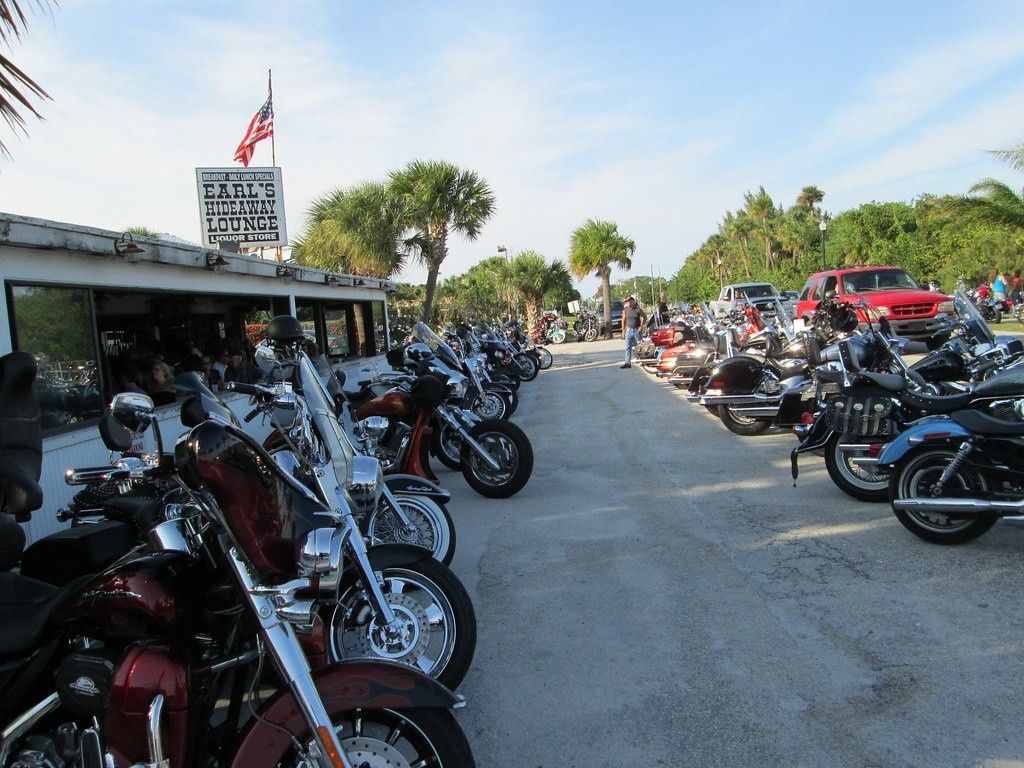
[{"xmin": 855, "ymin": 273, "xmax": 875, "ymax": 289}]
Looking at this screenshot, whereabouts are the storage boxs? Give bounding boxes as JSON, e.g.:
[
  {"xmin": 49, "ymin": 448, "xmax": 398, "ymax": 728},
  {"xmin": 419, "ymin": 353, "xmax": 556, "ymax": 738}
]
[
  {"xmin": 704, "ymin": 357, "xmax": 764, "ymax": 391},
  {"xmin": 650, "ymin": 327, "xmax": 675, "ymax": 347}
]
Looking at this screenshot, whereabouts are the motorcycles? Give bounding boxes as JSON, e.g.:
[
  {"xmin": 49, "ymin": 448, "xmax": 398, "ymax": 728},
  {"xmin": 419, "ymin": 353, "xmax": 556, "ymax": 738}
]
[
  {"xmin": 849, "ymin": 361, "xmax": 1024, "ymax": 544},
  {"xmin": 58, "ymin": 317, "xmax": 477, "ymax": 692},
  {"xmin": 633, "ymin": 301, "xmax": 905, "ymax": 435},
  {"xmin": 0, "ymin": 393, "xmax": 477, "ymax": 768},
  {"xmin": 544, "ymin": 313, "xmax": 568, "ymax": 344},
  {"xmin": 337, "ymin": 313, "xmax": 552, "ymax": 501},
  {"xmin": 574, "ymin": 310, "xmax": 598, "ymax": 342},
  {"xmin": 962, "ymin": 287, "xmax": 1024, "ymax": 324},
  {"xmin": 791, "ymin": 290, "xmax": 1024, "ymax": 502}
]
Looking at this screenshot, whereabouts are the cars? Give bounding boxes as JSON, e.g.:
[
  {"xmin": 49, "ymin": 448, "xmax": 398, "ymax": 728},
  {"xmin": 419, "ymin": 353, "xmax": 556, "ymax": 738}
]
[{"xmin": 777, "ymin": 290, "xmax": 801, "ymax": 308}]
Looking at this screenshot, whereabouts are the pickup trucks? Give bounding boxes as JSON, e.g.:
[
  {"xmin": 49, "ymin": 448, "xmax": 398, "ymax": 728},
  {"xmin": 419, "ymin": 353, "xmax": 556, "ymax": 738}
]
[{"xmin": 708, "ymin": 282, "xmax": 787, "ymax": 320}]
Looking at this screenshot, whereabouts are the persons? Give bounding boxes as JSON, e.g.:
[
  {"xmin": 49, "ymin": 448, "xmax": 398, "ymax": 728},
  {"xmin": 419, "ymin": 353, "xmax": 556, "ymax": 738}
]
[
  {"xmin": 958, "ymin": 277, "xmax": 966, "ymax": 294},
  {"xmin": 835, "ymin": 276, "xmax": 857, "ymax": 295},
  {"xmin": 658, "ymin": 293, "xmax": 671, "ymax": 325},
  {"xmin": 1011, "ymin": 268, "xmax": 1022, "ymax": 309},
  {"xmin": 102, "ymin": 339, "xmax": 333, "ymax": 406},
  {"xmin": 929, "ymin": 280, "xmax": 943, "ymax": 294},
  {"xmin": 918, "ymin": 281, "xmax": 930, "ymax": 291},
  {"xmin": 988, "ymin": 268, "xmax": 1010, "ymax": 315},
  {"xmin": 734, "ymin": 290, "xmax": 744, "ymax": 299},
  {"xmin": 620, "ymin": 297, "xmax": 647, "ymax": 369}
]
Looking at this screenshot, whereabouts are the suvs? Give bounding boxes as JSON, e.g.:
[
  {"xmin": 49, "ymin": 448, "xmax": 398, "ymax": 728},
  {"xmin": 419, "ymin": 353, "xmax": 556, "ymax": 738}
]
[
  {"xmin": 797, "ymin": 266, "xmax": 955, "ymax": 346},
  {"xmin": 597, "ymin": 300, "xmax": 625, "ymax": 335}
]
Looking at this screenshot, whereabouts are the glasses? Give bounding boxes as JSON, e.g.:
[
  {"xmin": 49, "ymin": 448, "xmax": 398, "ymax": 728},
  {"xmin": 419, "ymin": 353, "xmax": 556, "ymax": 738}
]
[{"xmin": 203, "ymin": 362, "xmax": 212, "ymax": 365}]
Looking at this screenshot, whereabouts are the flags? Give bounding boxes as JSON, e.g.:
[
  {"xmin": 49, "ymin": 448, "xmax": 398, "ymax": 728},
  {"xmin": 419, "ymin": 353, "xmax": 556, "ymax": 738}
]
[{"xmin": 234, "ymin": 92, "xmax": 273, "ymax": 167}]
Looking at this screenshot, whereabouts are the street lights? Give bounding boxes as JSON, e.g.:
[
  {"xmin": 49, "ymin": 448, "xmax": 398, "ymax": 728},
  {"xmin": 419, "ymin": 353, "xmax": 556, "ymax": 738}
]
[{"xmin": 819, "ymin": 220, "xmax": 827, "ymax": 271}]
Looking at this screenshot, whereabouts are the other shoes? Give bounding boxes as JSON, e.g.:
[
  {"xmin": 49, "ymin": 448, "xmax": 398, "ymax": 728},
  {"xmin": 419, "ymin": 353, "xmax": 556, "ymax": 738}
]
[{"xmin": 620, "ymin": 364, "xmax": 631, "ymax": 368}]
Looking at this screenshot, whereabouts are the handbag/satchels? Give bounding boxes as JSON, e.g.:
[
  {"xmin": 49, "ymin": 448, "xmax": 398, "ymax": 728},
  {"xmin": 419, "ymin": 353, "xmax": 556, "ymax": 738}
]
[{"xmin": 648, "ymin": 312, "xmax": 662, "ymax": 327}]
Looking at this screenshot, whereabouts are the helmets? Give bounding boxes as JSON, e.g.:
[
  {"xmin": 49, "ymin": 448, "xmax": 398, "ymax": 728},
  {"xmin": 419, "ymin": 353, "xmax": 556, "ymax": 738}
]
[
  {"xmin": 456, "ymin": 328, "xmax": 467, "ymax": 338},
  {"xmin": 403, "ymin": 343, "xmax": 435, "ymax": 365},
  {"xmin": 266, "ymin": 316, "xmax": 306, "ymax": 340},
  {"xmin": 509, "ymin": 321, "xmax": 518, "ymax": 326},
  {"xmin": 825, "ymin": 290, "xmax": 836, "ymax": 300},
  {"xmin": 838, "ymin": 310, "xmax": 859, "ymax": 331}
]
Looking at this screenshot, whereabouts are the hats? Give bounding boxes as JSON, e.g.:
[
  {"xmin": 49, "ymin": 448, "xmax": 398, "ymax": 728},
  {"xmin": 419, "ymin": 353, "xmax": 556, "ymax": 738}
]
[
  {"xmin": 225, "ymin": 349, "xmax": 241, "ymax": 356},
  {"xmin": 624, "ymin": 297, "xmax": 634, "ymax": 303}
]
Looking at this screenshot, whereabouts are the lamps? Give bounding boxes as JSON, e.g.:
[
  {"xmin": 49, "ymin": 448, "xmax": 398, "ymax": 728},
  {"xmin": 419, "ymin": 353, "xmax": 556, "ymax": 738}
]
[
  {"xmin": 113, "ymin": 234, "xmax": 145, "ymax": 265},
  {"xmin": 325, "ymin": 273, "xmax": 340, "ymax": 289},
  {"xmin": 276, "ymin": 261, "xmax": 292, "ymax": 284},
  {"xmin": 349, "ymin": 278, "xmax": 366, "ymax": 288},
  {"xmin": 204, "ymin": 249, "xmax": 229, "ymax": 276},
  {"xmin": 380, "ymin": 282, "xmax": 392, "ymax": 292}
]
